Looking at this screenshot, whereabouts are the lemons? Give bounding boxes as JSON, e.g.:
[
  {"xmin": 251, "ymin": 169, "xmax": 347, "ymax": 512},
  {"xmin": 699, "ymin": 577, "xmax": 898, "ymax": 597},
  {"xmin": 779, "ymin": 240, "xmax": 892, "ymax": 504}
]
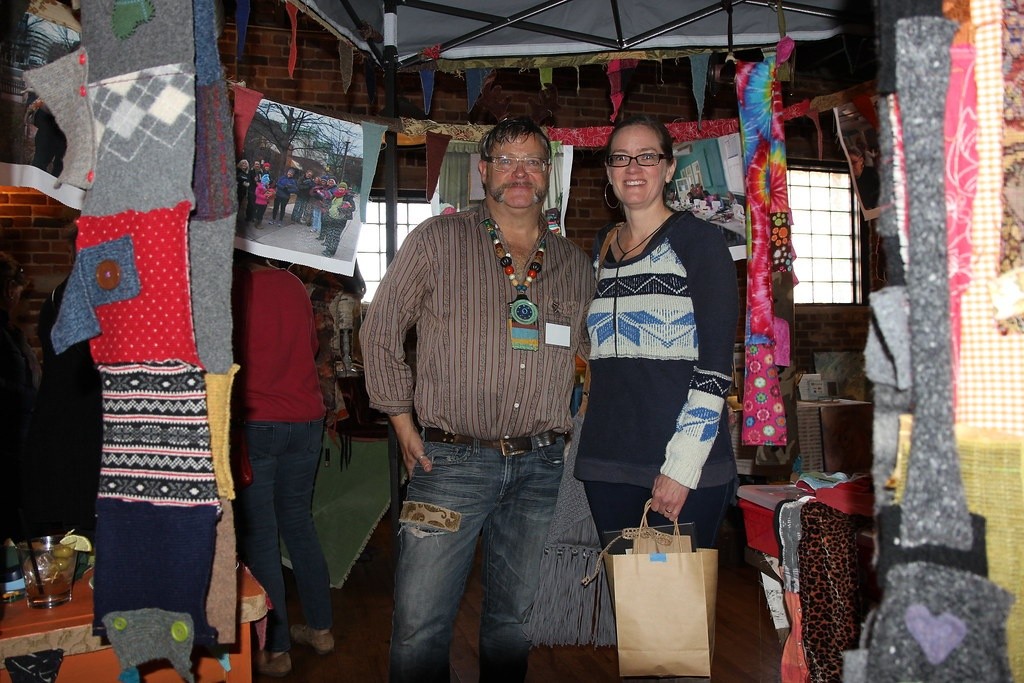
[{"xmin": 60, "ymin": 534, "xmax": 91, "ymax": 551}]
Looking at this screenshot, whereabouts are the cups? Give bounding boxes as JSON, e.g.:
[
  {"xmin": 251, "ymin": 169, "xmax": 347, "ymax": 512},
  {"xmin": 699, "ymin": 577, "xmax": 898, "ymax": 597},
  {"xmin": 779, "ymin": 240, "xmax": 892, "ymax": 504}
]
[{"xmin": 13, "ymin": 535, "xmax": 78, "ymax": 610}]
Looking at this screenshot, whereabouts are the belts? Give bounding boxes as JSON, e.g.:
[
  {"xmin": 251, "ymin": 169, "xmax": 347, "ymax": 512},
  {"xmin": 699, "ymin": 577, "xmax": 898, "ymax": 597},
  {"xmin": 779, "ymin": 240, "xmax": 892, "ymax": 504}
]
[{"xmin": 425, "ymin": 427, "xmax": 557, "ymax": 457}]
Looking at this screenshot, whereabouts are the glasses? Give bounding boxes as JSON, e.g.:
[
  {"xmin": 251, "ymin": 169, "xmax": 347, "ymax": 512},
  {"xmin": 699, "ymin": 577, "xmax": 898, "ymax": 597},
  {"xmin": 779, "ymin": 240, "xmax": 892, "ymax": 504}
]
[
  {"xmin": 484, "ymin": 156, "xmax": 551, "ymax": 173},
  {"xmin": 606, "ymin": 153, "xmax": 671, "ymax": 167}
]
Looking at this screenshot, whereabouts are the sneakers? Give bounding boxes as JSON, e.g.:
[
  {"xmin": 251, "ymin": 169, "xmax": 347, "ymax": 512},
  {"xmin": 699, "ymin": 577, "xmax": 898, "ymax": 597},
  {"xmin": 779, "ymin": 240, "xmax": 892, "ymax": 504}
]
[
  {"xmin": 290, "ymin": 623, "xmax": 335, "ymax": 655},
  {"xmin": 251, "ymin": 649, "xmax": 291, "ymax": 678}
]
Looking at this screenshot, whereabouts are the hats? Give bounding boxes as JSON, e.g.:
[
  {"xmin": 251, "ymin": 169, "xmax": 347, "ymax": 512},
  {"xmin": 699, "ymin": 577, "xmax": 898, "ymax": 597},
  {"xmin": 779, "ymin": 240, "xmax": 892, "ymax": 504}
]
[
  {"xmin": 289, "ymin": 168, "xmax": 295, "ymax": 175},
  {"xmin": 263, "ymin": 163, "xmax": 270, "ymax": 168},
  {"xmin": 339, "ymin": 182, "xmax": 347, "ymax": 190},
  {"xmin": 321, "ymin": 175, "xmax": 328, "ymax": 182},
  {"xmin": 260, "ymin": 174, "xmax": 270, "ymax": 184},
  {"xmin": 329, "ymin": 176, "xmax": 338, "ymax": 184}
]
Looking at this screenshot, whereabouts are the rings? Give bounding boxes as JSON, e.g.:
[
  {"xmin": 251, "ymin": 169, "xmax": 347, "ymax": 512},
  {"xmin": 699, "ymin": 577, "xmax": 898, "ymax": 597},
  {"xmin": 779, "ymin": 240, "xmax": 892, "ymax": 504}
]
[
  {"xmin": 666, "ymin": 509, "xmax": 672, "ymax": 513},
  {"xmin": 418, "ymin": 454, "xmax": 427, "ymax": 461}
]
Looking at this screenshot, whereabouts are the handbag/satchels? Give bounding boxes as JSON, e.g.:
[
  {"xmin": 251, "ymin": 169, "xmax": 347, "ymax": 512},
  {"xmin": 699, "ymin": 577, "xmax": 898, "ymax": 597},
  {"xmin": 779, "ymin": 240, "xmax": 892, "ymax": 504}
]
[
  {"xmin": 526, "ymin": 411, "xmax": 617, "ymax": 648},
  {"xmin": 603, "ymin": 498, "xmax": 718, "ymax": 677}
]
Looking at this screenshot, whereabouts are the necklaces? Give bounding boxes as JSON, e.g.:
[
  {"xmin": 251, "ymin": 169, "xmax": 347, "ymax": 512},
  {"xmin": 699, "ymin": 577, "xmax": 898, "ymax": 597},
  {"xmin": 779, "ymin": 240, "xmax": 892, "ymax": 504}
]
[
  {"xmin": 485, "ymin": 221, "xmax": 548, "ymax": 351},
  {"xmin": 617, "ymin": 221, "xmax": 665, "ymax": 255}
]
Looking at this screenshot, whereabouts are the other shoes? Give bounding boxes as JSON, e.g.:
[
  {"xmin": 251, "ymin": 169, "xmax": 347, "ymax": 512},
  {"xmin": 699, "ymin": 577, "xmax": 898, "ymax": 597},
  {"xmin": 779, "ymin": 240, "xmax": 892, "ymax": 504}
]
[
  {"xmin": 269, "ymin": 219, "xmax": 277, "ymax": 225},
  {"xmin": 278, "ymin": 220, "xmax": 282, "ymax": 227},
  {"xmin": 245, "ymin": 215, "xmax": 256, "ymax": 222},
  {"xmin": 290, "ymin": 215, "xmax": 334, "ymax": 258},
  {"xmin": 256, "ymin": 223, "xmax": 263, "ymax": 229}
]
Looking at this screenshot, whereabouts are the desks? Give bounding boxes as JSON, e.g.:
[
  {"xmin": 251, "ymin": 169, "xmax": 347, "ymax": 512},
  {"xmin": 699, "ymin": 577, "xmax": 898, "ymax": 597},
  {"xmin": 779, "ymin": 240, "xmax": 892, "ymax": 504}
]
[
  {"xmin": 801, "ymin": 398, "xmax": 875, "ymax": 473},
  {"xmin": 326, "ymin": 373, "xmax": 402, "ymax": 562},
  {"xmin": 0, "ymin": 548, "xmax": 269, "ymax": 683}
]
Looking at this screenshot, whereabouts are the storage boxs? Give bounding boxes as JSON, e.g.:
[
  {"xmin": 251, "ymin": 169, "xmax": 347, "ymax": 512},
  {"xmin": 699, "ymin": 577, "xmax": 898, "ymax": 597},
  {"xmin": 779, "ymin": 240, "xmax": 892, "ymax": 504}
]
[{"xmin": 735, "ymin": 484, "xmax": 815, "ymax": 559}]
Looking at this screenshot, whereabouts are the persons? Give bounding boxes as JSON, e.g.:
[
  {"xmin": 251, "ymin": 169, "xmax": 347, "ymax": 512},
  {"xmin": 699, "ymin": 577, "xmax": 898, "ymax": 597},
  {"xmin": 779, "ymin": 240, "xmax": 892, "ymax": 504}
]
[
  {"xmin": 0, "ymin": 252, "xmax": 103, "ymax": 540},
  {"xmin": 237, "ymin": 160, "xmax": 356, "ymax": 257},
  {"xmin": 688, "ymin": 184, "xmax": 737, "ymax": 210},
  {"xmin": 19, "ymin": 86, "xmax": 68, "ymax": 178},
  {"xmin": 358, "ymin": 118, "xmax": 593, "ymax": 683},
  {"xmin": 574, "ymin": 116, "xmax": 738, "ymax": 682},
  {"xmin": 231, "ymin": 249, "xmax": 334, "ymax": 675}
]
